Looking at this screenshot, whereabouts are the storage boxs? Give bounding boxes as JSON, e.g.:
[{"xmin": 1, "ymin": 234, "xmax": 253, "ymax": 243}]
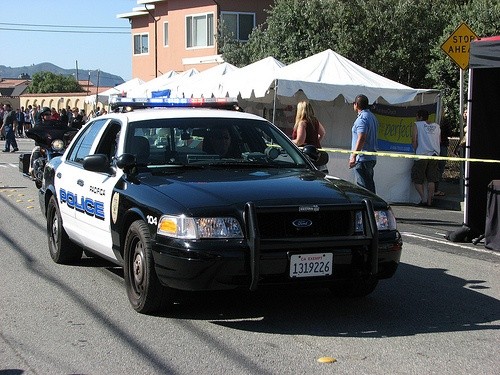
[{"xmin": 19, "ymin": 153, "xmax": 31, "ymax": 173}]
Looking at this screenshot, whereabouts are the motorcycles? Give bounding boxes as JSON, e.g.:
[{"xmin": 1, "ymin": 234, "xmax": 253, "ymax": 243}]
[{"xmin": 18, "ymin": 121, "xmax": 78, "ymax": 189}]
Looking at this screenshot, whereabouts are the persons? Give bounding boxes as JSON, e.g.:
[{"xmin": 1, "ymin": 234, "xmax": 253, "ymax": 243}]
[
  {"xmin": 349, "ymin": 94, "xmax": 378, "ymax": 194},
  {"xmin": 459, "ymin": 110, "xmax": 467, "ymax": 165},
  {"xmin": 291, "ymin": 101, "xmax": 325, "ymax": 149},
  {"xmin": 0, "ymin": 104, "xmax": 18, "ymax": 152},
  {"xmin": 0, "ymin": 103, "xmax": 127, "ymax": 140},
  {"xmin": 25, "ymin": 107, "xmax": 79, "ymax": 181},
  {"xmin": 209, "ymin": 128, "xmax": 242, "ymax": 159},
  {"xmin": 412, "ymin": 109, "xmax": 449, "ymax": 206}
]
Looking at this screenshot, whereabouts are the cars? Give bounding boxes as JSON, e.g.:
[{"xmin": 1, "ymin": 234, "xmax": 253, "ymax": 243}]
[{"xmin": 18, "ymin": 96, "xmax": 404, "ymax": 315}]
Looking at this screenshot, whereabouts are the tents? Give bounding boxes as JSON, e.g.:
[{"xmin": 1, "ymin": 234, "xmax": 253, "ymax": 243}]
[
  {"xmin": 463, "ymin": 40, "xmax": 500, "ymax": 225},
  {"xmin": 85, "ymin": 48, "xmax": 443, "ymax": 147}
]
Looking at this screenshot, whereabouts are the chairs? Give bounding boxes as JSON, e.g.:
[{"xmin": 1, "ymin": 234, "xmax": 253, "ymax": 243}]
[{"xmin": 125, "ymin": 136, "xmax": 150, "ymax": 164}]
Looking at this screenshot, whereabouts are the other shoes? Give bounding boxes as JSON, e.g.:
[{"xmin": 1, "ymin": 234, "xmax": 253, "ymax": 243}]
[
  {"xmin": 11, "ymin": 148, "xmax": 18, "ymax": 153},
  {"xmin": 419, "ymin": 200, "xmax": 431, "ymax": 208},
  {"xmin": 2, "ymin": 149, "xmax": 10, "ymax": 152}
]
